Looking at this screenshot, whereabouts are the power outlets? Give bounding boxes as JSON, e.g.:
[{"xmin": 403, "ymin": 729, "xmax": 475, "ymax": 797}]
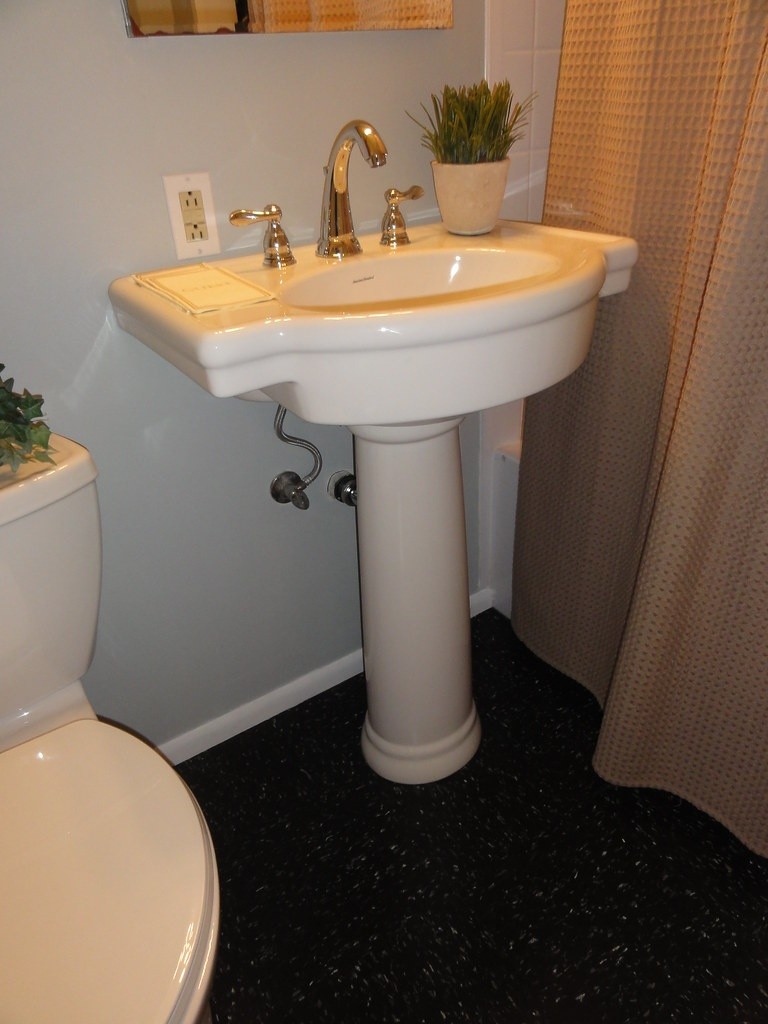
[{"xmin": 160, "ymin": 170, "xmax": 222, "ymax": 261}]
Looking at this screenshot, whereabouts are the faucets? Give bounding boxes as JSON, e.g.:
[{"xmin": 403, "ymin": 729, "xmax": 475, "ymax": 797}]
[{"xmin": 314, "ymin": 115, "xmax": 390, "ymax": 259}]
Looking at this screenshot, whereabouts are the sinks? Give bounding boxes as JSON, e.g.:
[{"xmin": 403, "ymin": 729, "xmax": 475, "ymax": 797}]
[{"xmin": 109, "ymin": 207, "xmax": 641, "ymax": 430}]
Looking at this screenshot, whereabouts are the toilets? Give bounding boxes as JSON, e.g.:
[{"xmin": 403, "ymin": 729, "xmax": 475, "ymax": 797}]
[{"xmin": 0, "ymin": 414, "xmax": 225, "ymax": 1024}]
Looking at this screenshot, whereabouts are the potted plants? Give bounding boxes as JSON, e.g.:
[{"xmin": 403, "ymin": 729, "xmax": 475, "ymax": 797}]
[{"xmin": 404, "ymin": 76, "xmax": 539, "ymax": 235}]
